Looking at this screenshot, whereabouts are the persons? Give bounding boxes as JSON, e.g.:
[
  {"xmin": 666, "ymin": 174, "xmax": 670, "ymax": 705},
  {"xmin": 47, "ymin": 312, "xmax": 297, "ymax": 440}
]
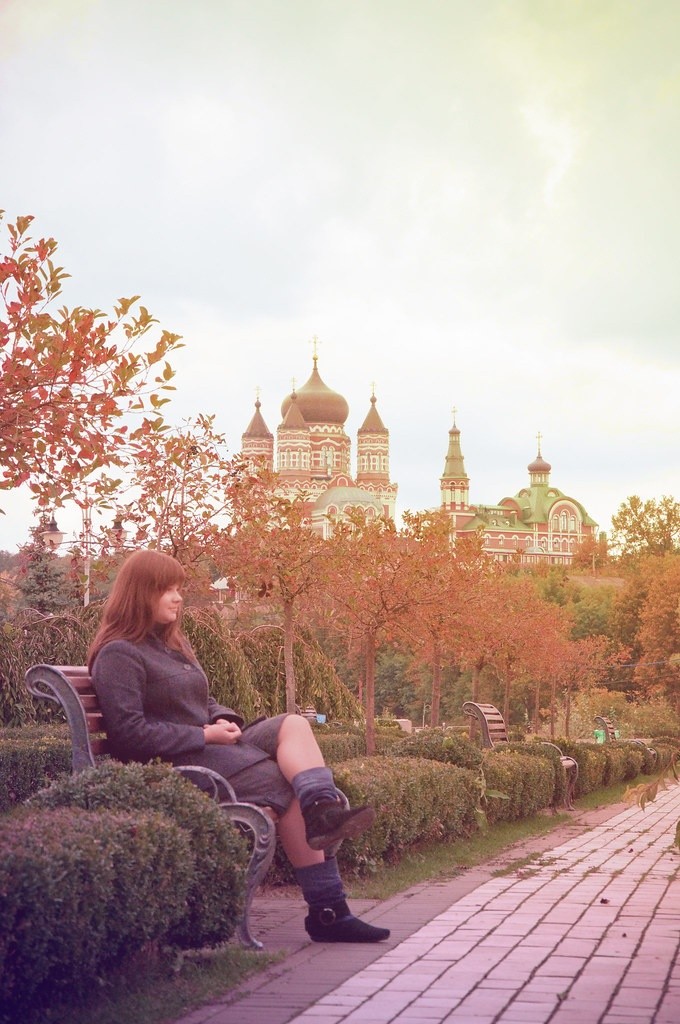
[{"xmin": 89, "ymin": 549, "xmax": 390, "ymax": 942}]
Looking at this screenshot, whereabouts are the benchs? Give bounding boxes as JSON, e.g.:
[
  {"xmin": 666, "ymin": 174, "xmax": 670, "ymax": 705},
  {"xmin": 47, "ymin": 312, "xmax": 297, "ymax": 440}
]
[
  {"xmin": 592, "ymin": 715, "xmax": 657, "ymax": 766},
  {"xmin": 24, "ymin": 661, "xmax": 280, "ymax": 954},
  {"xmin": 294, "ymin": 703, "xmax": 343, "ymax": 729},
  {"xmin": 462, "ymin": 701, "xmax": 578, "ymax": 813}
]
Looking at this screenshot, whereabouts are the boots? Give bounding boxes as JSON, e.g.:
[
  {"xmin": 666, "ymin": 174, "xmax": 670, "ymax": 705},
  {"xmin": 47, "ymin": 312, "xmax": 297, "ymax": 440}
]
[
  {"xmin": 293, "ymin": 855, "xmax": 390, "ymax": 943},
  {"xmin": 290, "ymin": 767, "xmax": 375, "ymax": 850}
]
